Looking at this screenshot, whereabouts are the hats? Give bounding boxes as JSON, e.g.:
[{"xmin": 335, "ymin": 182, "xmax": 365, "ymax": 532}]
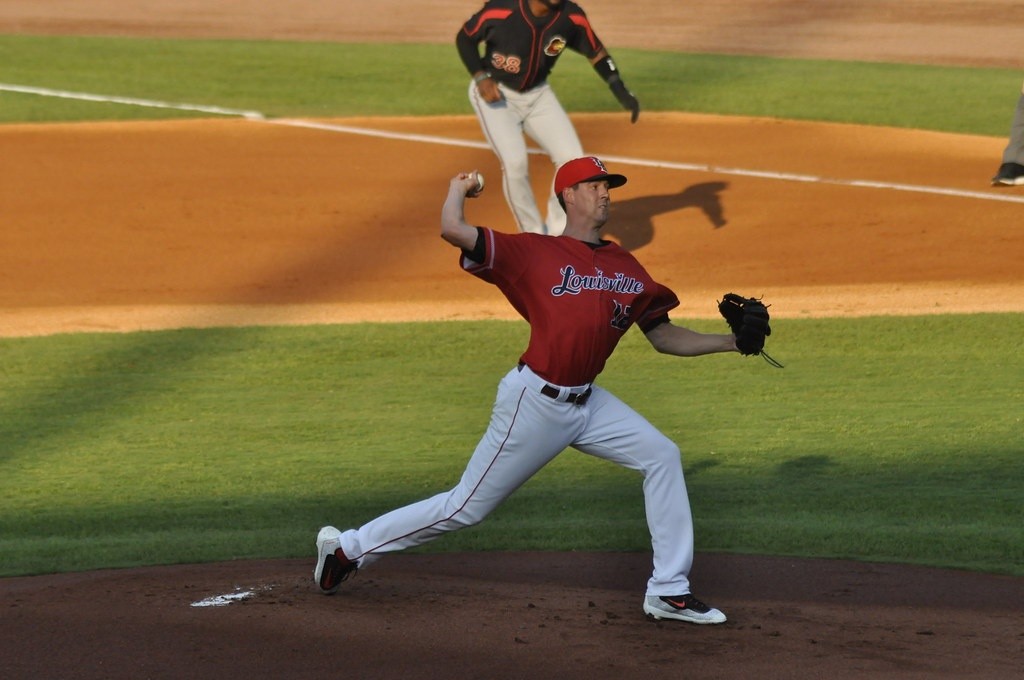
[{"xmin": 554, "ymin": 156, "xmax": 627, "ymax": 196}]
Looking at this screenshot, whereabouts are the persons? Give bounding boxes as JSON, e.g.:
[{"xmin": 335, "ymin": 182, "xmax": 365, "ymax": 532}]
[
  {"xmin": 456, "ymin": 0, "xmax": 640, "ymax": 237},
  {"xmin": 315, "ymin": 157, "xmax": 772, "ymax": 625},
  {"xmin": 991, "ymin": 83, "xmax": 1024, "ymax": 186}
]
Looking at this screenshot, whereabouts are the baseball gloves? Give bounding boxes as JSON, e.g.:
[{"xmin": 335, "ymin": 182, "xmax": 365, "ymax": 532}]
[{"xmin": 718, "ymin": 293, "xmax": 771, "ymax": 356}]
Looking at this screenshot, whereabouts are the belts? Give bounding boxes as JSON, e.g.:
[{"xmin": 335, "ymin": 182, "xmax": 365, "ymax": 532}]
[{"xmin": 518, "ymin": 362, "xmax": 592, "ymax": 406}]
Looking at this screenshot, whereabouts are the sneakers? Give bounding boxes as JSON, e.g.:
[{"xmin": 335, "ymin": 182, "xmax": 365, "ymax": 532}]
[
  {"xmin": 313, "ymin": 525, "xmax": 360, "ymax": 593},
  {"xmin": 643, "ymin": 592, "xmax": 727, "ymax": 624}
]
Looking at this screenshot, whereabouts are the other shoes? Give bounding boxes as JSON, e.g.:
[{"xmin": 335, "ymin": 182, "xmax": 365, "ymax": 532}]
[{"xmin": 991, "ymin": 163, "xmax": 1024, "ymax": 185}]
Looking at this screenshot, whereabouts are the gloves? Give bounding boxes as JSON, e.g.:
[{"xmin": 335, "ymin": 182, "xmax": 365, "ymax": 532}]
[{"xmin": 609, "ymin": 80, "xmax": 639, "ymax": 123}]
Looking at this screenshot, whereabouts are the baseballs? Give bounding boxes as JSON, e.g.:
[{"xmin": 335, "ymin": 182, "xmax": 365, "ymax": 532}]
[{"xmin": 467, "ymin": 172, "xmax": 485, "ymax": 192}]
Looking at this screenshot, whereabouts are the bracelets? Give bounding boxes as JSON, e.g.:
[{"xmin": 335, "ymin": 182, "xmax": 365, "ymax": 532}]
[{"xmin": 476, "ymin": 72, "xmax": 494, "ymax": 82}]
